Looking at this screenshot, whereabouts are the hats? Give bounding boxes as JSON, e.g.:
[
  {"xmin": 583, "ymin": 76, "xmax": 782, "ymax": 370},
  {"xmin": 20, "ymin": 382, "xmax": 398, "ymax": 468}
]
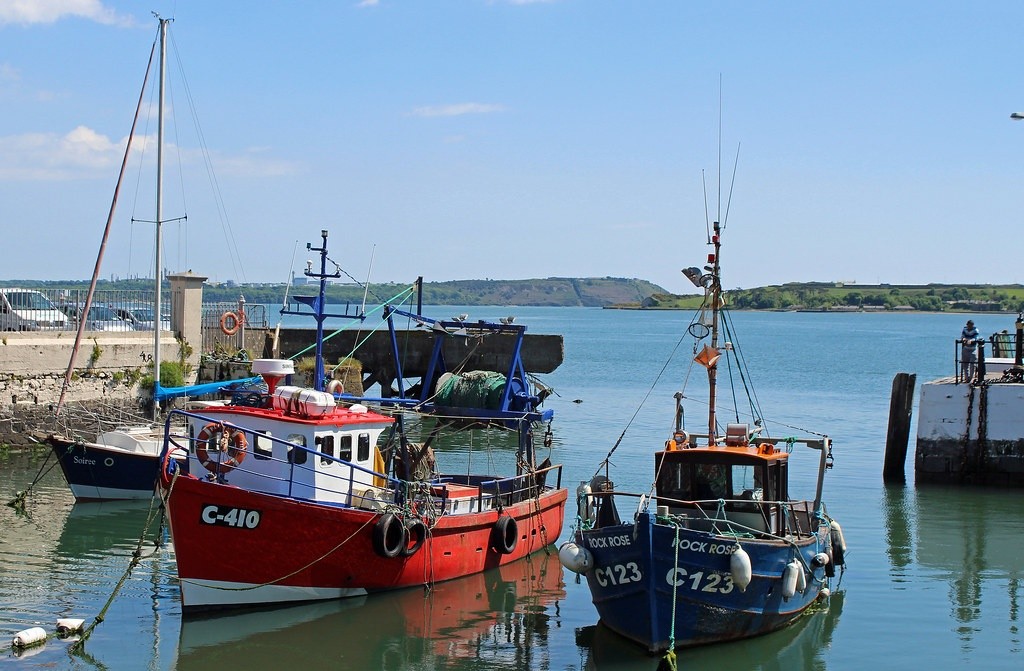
[{"xmin": 966, "ymin": 320, "xmax": 974, "ymax": 325}]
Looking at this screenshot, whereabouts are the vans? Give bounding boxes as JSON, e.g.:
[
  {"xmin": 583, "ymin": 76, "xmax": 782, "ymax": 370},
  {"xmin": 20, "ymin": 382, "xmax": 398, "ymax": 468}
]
[{"xmin": 0, "ymin": 288, "xmax": 70, "ymax": 331}]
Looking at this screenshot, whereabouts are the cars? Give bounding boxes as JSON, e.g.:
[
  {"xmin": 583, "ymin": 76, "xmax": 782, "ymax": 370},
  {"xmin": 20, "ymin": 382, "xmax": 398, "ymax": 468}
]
[
  {"xmin": 110, "ymin": 307, "xmax": 170, "ymax": 332},
  {"xmin": 54, "ymin": 304, "xmax": 135, "ymax": 332}
]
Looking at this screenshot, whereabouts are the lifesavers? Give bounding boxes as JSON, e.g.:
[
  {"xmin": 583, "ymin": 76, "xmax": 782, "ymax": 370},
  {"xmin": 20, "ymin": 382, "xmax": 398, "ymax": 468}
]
[
  {"xmin": 326, "ymin": 379, "xmax": 345, "ymax": 396},
  {"xmin": 195, "ymin": 419, "xmax": 249, "ymax": 474},
  {"xmin": 220, "ymin": 311, "xmax": 240, "ymax": 335},
  {"xmin": 492, "ymin": 515, "xmax": 518, "ymax": 554},
  {"xmin": 371, "ymin": 513, "xmax": 427, "ymax": 559}
]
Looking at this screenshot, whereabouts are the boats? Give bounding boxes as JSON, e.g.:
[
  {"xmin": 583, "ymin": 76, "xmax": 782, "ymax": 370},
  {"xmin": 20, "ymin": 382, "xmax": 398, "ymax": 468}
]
[
  {"xmin": 558, "ymin": 72, "xmax": 846, "ymax": 652},
  {"xmin": 159, "ymin": 228, "xmax": 567, "ymax": 606},
  {"xmin": 51, "ymin": 12, "xmax": 306, "ymax": 502}
]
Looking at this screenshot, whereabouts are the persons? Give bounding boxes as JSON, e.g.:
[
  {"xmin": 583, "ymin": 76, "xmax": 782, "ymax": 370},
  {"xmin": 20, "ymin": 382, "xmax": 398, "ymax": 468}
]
[
  {"xmin": 961, "ymin": 320, "xmax": 979, "ymax": 380},
  {"xmin": 63, "ymin": 304, "xmax": 83, "ymax": 322}
]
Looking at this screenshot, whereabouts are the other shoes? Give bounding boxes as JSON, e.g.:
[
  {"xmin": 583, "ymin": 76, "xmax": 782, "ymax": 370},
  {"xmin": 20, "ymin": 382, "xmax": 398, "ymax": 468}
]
[{"xmin": 964, "ymin": 377, "xmax": 971, "ymax": 383}]
